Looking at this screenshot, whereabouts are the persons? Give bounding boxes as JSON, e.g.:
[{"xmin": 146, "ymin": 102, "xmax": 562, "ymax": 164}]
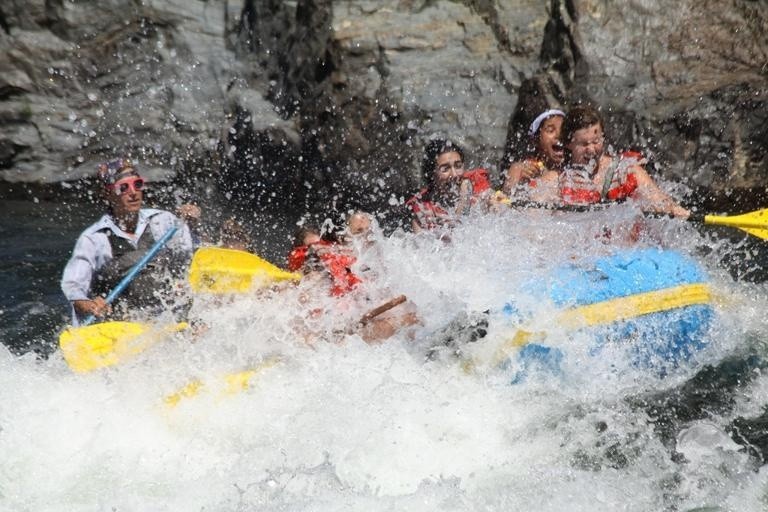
[
  {"xmin": 501, "ymin": 107, "xmax": 689, "ymax": 246},
  {"xmin": 290, "ymin": 204, "xmax": 419, "ymax": 347},
  {"xmin": 175, "ymin": 108, "xmax": 249, "ymax": 252},
  {"xmin": 59, "ymin": 159, "xmax": 201, "ymax": 323},
  {"xmin": 408, "ymin": 139, "xmax": 507, "ymax": 236}
]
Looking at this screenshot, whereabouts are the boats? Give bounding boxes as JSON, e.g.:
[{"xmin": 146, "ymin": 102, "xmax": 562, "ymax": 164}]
[{"xmin": 460, "ymin": 248, "xmax": 713, "ymax": 403}]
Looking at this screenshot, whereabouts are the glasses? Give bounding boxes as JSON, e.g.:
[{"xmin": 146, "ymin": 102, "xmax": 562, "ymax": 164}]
[{"xmin": 105, "ymin": 177, "xmax": 144, "ymax": 194}]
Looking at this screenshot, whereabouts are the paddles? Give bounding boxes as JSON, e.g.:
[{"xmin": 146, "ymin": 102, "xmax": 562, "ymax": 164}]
[
  {"xmin": 59, "ymin": 321, "xmax": 193, "ymax": 374},
  {"xmin": 495, "ymin": 190, "xmax": 768, "ymax": 241},
  {"xmin": 189, "ymin": 247, "xmax": 307, "ymax": 294},
  {"xmin": 169, "ymin": 296, "xmax": 406, "ymax": 410}
]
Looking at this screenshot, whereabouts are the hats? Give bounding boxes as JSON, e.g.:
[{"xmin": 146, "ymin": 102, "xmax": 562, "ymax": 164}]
[{"xmin": 97, "ymin": 157, "xmax": 140, "ymax": 185}]
